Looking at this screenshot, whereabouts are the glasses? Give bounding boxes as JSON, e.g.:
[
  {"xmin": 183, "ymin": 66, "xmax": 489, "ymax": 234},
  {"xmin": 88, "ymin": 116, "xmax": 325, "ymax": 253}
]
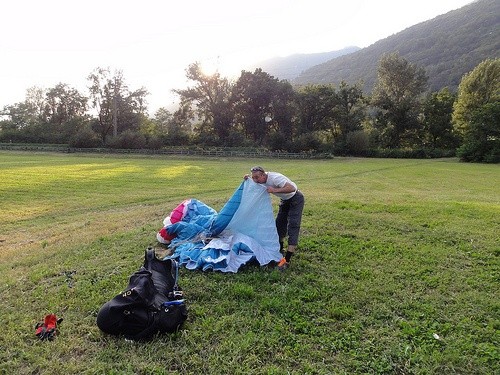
[{"xmin": 251, "ymin": 168, "xmax": 264, "ymax": 173}]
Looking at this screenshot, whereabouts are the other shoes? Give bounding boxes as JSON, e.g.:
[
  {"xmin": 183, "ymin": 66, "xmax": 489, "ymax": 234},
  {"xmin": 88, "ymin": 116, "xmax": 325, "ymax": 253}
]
[{"xmin": 277, "ymin": 257, "xmax": 288, "ymax": 267}]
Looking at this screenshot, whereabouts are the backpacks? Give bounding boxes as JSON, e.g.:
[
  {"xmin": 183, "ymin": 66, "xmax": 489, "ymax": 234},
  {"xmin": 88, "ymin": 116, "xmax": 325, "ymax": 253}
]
[{"xmin": 144, "ymin": 247, "xmax": 186, "ymax": 311}]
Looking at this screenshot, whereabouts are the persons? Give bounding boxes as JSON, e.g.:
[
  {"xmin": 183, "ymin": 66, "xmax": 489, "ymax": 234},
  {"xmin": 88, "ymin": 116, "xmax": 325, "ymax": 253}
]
[{"xmin": 244, "ymin": 166, "xmax": 305, "ymax": 270}]
